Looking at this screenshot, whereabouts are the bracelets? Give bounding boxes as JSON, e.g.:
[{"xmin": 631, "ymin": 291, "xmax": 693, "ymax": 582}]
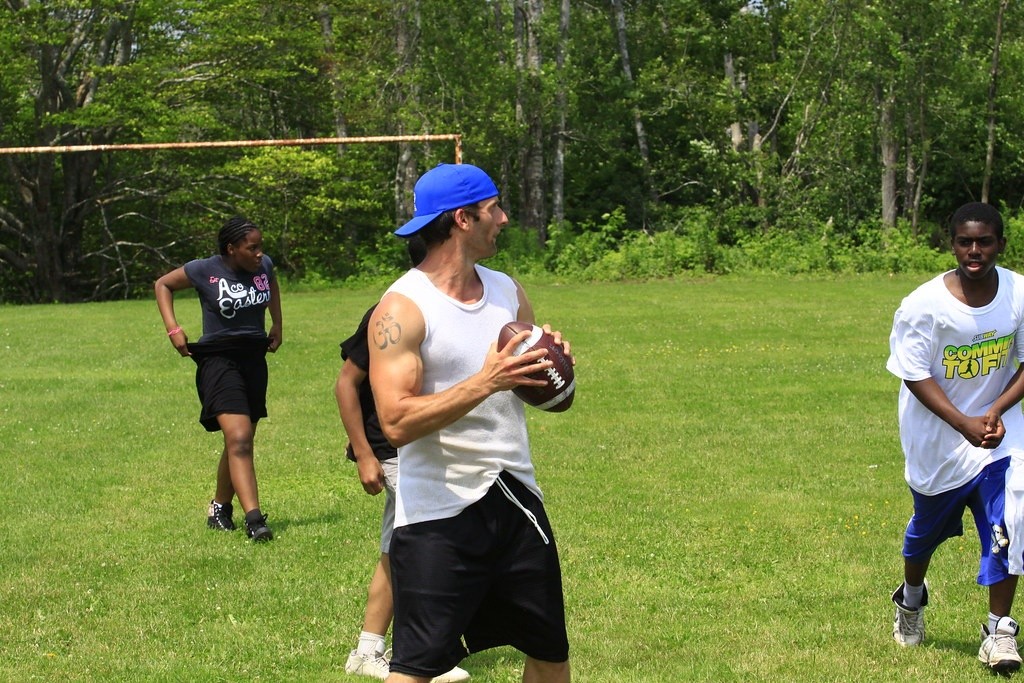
[{"xmin": 167, "ymin": 327, "xmax": 180, "ymax": 335}]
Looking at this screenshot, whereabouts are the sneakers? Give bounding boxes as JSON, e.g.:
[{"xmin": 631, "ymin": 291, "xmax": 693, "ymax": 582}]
[
  {"xmin": 207, "ymin": 498, "xmax": 236, "ymax": 532},
  {"xmin": 979, "ymin": 617, "xmax": 1022, "ymax": 675},
  {"xmin": 429, "ymin": 666, "xmax": 471, "ymax": 683},
  {"xmin": 245, "ymin": 512, "xmax": 273, "ymax": 543},
  {"xmin": 890, "ymin": 578, "xmax": 930, "ymax": 647},
  {"xmin": 345, "ymin": 640, "xmax": 394, "ymax": 680}
]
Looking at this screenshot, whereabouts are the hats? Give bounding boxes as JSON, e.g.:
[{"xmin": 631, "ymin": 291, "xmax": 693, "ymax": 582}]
[{"xmin": 394, "ymin": 164, "xmax": 498, "ymax": 238}]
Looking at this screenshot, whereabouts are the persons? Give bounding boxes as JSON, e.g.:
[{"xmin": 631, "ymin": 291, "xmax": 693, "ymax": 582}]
[
  {"xmin": 366, "ymin": 163, "xmax": 576, "ymax": 683},
  {"xmin": 154, "ymin": 215, "xmax": 282, "ymax": 540},
  {"xmin": 886, "ymin": 205, "xmax": 1024, "ymax": 668},
  {"xmin": 334, "ymin": 303, "xmax": 472, "ymax": 683}
]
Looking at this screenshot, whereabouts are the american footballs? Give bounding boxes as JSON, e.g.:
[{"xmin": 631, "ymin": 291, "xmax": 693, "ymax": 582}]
[{"xmin": 498, "ymin": 323, "xmax": 578, "ymax": 415}]
